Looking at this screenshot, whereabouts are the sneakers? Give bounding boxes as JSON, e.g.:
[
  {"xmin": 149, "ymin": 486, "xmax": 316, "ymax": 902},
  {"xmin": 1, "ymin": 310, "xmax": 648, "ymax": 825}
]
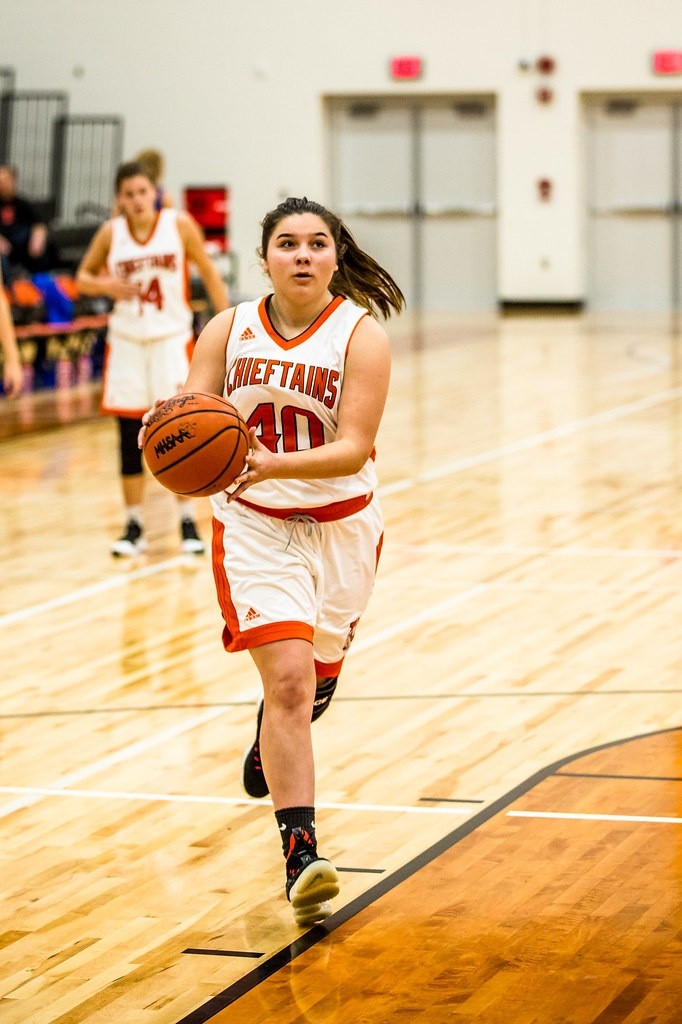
[
  {"xmin": 243, "ymin": 697, "xmax": 270, "ymax": 798},
  {"xmin": 180, "ymin": 517, "xmax": 206, "ymax": 555},
  {"xmin": 110, "ymin": 519, "xmax": 148, "ymax": 557},
  {"xmin": 284, "ymin": 825, "xmax": 339, "ymax": 928}
]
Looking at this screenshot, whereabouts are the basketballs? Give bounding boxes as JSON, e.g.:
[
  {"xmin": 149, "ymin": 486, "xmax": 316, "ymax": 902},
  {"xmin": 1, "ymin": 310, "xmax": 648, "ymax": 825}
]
[{"xmin": 143, "ymin": 392, "xmax": 250, "ymax": 498}]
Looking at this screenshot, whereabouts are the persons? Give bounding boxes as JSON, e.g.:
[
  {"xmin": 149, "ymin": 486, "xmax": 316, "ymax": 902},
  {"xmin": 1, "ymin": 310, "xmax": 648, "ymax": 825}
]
[
  {"xmin": 0, "ymin": 162, "xmax": 50, "ymax": 276},
  {"xmin": 139, "ymin": 195, "xmax": 408, "ymax": 926},
  {"xmin": 78, "ymin": 161, "xmax": 231, "ymax": 557},
  {"xmin": 0, "ymin": 274, "xmax": 27, "ymax": 403},
  {"xmin": 109, "ymin": 146, "xmax": 175, "ymax": 219}
]
